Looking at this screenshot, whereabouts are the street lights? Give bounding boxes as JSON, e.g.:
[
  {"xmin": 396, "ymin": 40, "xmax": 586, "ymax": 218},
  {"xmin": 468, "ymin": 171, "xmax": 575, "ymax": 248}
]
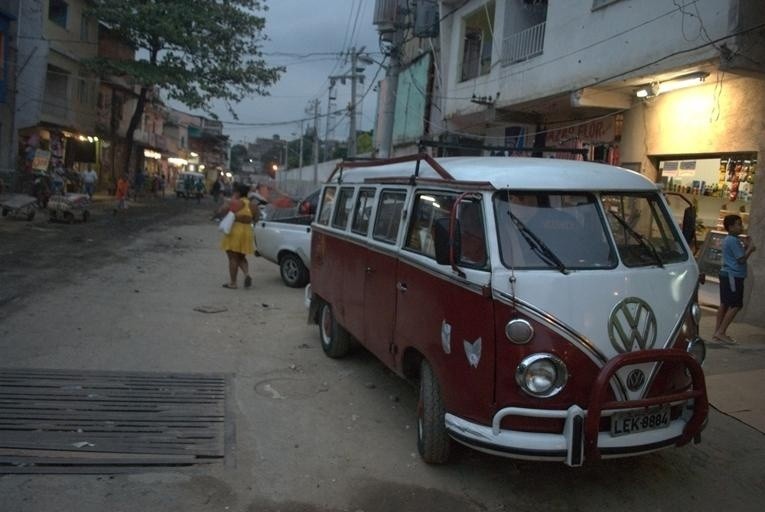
[
  {"xmin": 332, "ymin": 112, "xmax": 356, "ymax": 157},
  {"xmin": 357, "ymin": 55, "xmax": 401, "ymax": 156}
]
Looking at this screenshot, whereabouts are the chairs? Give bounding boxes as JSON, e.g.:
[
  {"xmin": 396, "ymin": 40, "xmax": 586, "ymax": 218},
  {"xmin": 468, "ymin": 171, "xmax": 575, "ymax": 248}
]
[
  {"xmin": 534, "ymin": 210, "xmax": 590, "ymax": 270},
  {"xmin": 454, "ymin": 204, "xmax": 512, "ymax": 267}
]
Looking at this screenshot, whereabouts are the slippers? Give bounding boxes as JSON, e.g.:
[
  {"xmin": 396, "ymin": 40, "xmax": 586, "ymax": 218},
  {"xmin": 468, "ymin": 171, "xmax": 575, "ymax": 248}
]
[
  {"xmin": 222, "ymin": 276, "xmax": 251, "ymax": 290},
  {"xmin": 713, "ymin": 334, "xmax": 741, "ymax": 346}
]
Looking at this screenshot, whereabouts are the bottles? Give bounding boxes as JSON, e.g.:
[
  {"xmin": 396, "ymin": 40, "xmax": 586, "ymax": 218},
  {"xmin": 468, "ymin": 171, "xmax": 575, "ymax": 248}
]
[{"xmin": 708, "ymin": 236, "xmax": 723, "ymax": 261}]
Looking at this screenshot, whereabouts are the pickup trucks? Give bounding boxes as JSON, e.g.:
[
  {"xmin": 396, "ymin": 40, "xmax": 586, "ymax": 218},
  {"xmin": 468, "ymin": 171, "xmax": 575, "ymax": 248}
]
[{"xmin": 253, "ymin": 212, "xmax": 314, "ymax": 287}]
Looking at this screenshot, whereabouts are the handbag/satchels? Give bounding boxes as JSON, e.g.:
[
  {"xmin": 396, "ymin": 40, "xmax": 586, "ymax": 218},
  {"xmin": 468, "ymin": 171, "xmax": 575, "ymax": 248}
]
[{"xmin": 218, "ymin": 212, "xmax": 236, "ymax": 234}]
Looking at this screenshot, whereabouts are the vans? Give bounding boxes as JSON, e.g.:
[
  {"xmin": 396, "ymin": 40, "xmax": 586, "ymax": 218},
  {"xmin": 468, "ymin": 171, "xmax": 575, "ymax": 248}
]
[
  {"xmin": 304, "ymin": 141, "xmax": 709, "ymax": 466},
  {"xmin": 175, "ymin": 171, "xmax": 205, "ymax": 198}
]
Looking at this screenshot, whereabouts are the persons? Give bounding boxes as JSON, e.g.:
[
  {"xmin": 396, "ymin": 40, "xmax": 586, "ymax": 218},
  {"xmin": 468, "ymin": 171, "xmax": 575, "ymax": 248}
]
[
  {"xmin": 143, "ymin": 168, "xmax": 149, "ymax": 176},
  {"xmin": 184, "ymin": 174, "xmax": 239, "ymax": 203},
  {"xmin": 114, "ymin": 172, "xmax": 130, "ymax": 210},
  {"xmin": 711, "ymin": 215, "xmax": 755, "ymax": 346},
  {"xmin": 160, "ymin": 174, "xmax": 165, "ymax": 199},
  {"xmin": 81, "ymin": 163, "xmax": 98, "ymax": 198},
  {"xmin": 134, "ymin": 169, "xmax": 145, "ymax": 203},
  {"xmin": 219, "ymin": 183, "xmax": 262, "ymax": 290}
]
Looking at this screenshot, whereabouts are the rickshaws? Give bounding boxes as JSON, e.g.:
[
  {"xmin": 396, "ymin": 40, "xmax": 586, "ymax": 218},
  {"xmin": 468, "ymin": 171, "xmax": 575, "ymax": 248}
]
[{"xmin": 48, "ymin": 193, "xmax": 134, "ymax": 223}]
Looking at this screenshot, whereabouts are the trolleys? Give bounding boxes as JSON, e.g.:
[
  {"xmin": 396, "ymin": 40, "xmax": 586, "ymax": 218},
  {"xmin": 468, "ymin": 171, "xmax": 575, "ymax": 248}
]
[{"xmin": 1, "ymin": 194, "xmax": 39, "ymax": 221}]
